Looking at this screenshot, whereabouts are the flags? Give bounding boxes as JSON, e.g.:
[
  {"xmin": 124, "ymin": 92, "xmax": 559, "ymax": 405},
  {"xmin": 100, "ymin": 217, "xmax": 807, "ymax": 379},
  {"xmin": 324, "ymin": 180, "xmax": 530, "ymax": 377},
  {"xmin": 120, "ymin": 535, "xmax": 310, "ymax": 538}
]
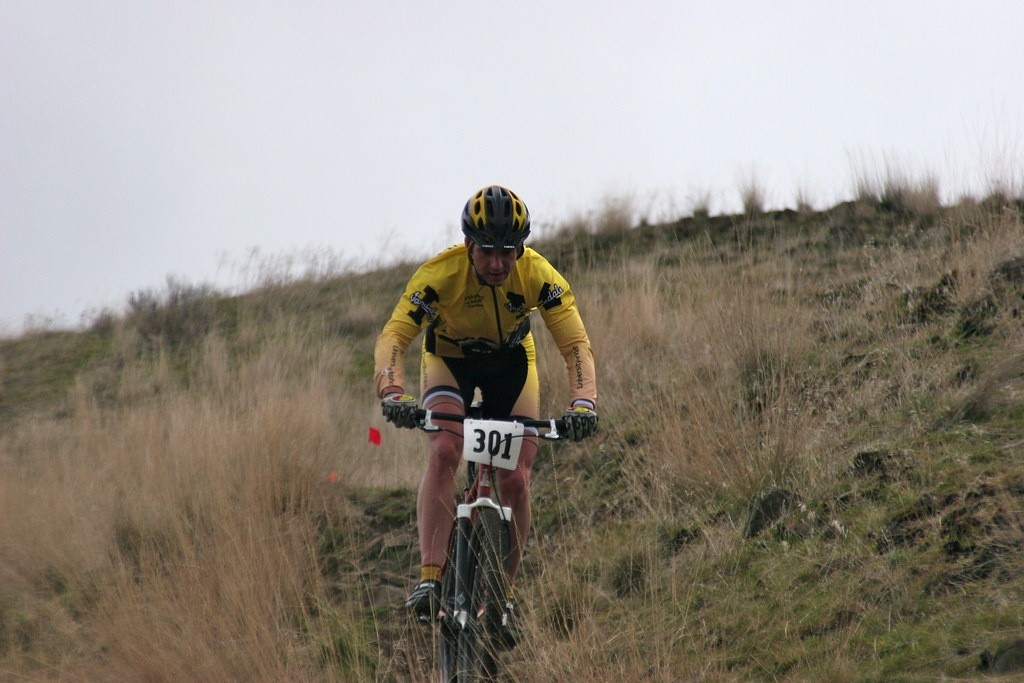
[{"xmin": 368, "ymin": 427, "xmax": 381, "ymax": 445}]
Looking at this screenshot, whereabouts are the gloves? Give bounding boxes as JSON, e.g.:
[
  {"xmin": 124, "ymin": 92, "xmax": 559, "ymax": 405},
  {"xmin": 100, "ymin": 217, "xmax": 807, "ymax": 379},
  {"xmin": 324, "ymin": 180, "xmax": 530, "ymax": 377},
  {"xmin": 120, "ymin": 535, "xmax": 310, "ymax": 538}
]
[
  {"xmin": 561, "ymin": 405, "xmax": 598, "ymax": 443},
  {"xmin": 381, "ymin": 390, "xmax": 417, "ymax": 429}
]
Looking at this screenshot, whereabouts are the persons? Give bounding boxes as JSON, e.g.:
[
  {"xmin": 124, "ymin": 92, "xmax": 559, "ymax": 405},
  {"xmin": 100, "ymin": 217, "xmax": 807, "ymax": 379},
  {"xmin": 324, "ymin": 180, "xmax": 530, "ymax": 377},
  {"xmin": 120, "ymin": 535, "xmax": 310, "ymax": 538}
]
[{"xmin": 374, "ymin": 185, "xmax": 598, "ymax": 648}]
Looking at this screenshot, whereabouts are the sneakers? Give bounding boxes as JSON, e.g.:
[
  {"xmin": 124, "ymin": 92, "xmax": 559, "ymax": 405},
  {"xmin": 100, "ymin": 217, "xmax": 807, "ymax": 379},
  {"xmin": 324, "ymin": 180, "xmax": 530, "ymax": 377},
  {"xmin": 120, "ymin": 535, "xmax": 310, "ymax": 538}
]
[
  {"xmin": 501, "ymin": 602, "xmax": 524, "ymax": 648},
  {"xmin": 408, "ymin": 580, "xmax": 442, "ymax": 618}
]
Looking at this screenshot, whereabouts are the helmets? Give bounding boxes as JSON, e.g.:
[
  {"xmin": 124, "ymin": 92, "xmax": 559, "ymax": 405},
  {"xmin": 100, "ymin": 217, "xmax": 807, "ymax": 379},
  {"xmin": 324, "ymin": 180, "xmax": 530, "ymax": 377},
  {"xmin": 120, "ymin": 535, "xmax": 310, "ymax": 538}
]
[{"xmin": 461, "ymin": 185, "xmax": 530, "ymax": 248}]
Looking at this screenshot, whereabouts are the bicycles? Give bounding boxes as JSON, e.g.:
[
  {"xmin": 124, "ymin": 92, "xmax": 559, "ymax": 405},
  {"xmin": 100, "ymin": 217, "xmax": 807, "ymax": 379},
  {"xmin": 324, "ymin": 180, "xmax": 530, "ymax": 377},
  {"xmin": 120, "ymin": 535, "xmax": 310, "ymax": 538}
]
[{"xmin": 409, "ymin": 407, "xmax": 566, "ymax": 683}]
